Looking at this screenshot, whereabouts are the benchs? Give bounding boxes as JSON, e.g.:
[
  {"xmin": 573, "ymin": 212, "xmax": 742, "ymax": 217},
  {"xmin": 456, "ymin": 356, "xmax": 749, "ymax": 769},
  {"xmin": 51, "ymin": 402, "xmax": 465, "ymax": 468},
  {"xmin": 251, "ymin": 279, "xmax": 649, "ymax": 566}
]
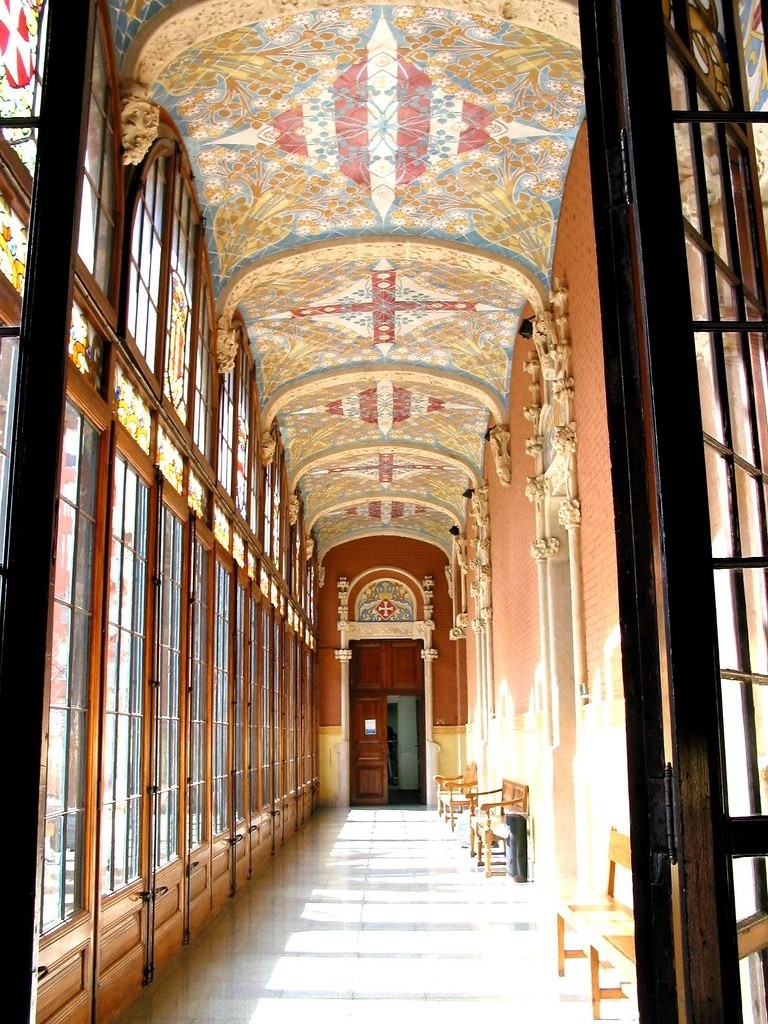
[
  {"xmin": 434, "ymin": 763, "xmax": 478, "ymax": 832},
  {"xmin": 555, "ymin": 827, "xmax": 635, "ymax": 1018},
  {"xmin": 465, "ymin": 778, "xmax": 529, "ymax": 876}
]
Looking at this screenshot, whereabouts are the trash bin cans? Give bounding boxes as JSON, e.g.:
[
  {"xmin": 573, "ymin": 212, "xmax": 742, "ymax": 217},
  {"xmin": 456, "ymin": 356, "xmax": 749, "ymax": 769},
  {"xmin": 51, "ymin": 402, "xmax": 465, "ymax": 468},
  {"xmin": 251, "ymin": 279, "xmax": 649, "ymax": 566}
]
[{"xmin": 504, "ymin": 813, "xmax": 527, "ymax": 882}]
